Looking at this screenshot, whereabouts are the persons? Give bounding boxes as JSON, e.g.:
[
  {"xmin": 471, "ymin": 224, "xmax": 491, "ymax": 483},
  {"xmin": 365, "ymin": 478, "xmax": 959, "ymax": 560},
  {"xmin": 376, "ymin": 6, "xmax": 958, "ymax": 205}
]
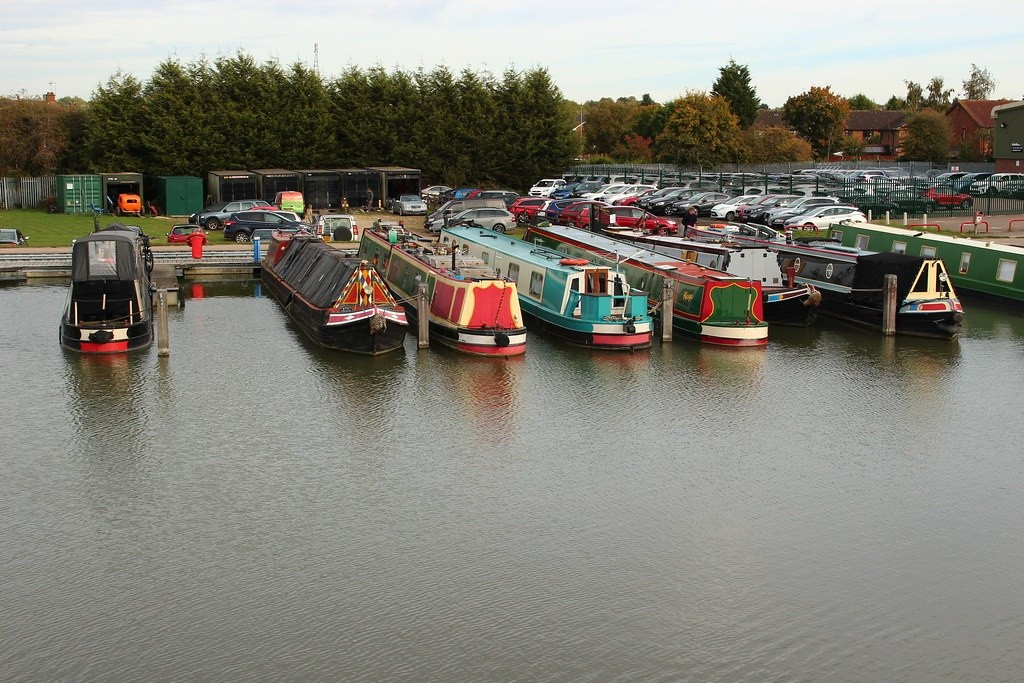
[
  {"xmin": 366, "ymin": 188, "xmax": 373, "ymax": 212},
  {"xmin": 681, "ymin": 207, "xmax": 698, "ymax": 238}
]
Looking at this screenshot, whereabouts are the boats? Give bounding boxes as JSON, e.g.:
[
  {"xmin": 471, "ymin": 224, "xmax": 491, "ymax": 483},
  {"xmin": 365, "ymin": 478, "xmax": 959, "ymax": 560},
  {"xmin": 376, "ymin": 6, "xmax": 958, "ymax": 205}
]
[
  {"xmin": 683, "ymin": 221, "xmax": 966, "ymax": 325},
  {"xmin": 591, "ymin": 209, "xmax": 823, "ymax": 330},
  {"xmin": 260, "ymin": 228, "xmax": 410, "ymax": 356},
  {"xmin": 416, "ymin": 209, "xmax": 655, "ymax": 350},
  {"xmin": 337, "ymin": 217, "xmax": 528, "ymax": 356},
  {"xmin": 59, "ymin": 213, "xmax": 154, "ymax": 355},
  {"xmin": 521, "ymin": 222, "xmax": 771, "ymax": 347},
  {"xmin": 826, "ymin": 221, "xmax": 1024, "ymax": 301}
]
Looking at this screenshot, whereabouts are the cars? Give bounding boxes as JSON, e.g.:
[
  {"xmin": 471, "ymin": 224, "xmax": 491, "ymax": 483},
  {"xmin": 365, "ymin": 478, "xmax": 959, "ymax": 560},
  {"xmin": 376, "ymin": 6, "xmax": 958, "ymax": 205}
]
[
  {"xmin": 0, "ymin": 228, "xmax": 30, "ymax": 248},
  {"xmin": 527, "ymin": 169, "xmax": 1024, "ymax": 232},
  {"xmin": 163, "ymin": 224, "xmax": 210, "ymax": 245},
  {"xmin": 421, "ymin": 185, "xmax": 679, "ymax": 237},
  {"xmin": 116, "ymin": 193, "xmax": 141, "ymax": 216}
]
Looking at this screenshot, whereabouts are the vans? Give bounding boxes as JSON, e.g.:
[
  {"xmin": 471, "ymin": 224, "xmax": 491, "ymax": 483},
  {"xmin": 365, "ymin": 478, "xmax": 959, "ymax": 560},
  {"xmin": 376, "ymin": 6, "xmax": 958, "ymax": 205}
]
[
  {"xmin": 311, "ymin": 213, "xmax": 359, "ymax": 241},
  {"xmin": 271, "ymin": 190, "xmax": 305, "ymax": 219},
  {"xmin": 423, "ymin": 200, "xmax": 508, "ymax": 229}
]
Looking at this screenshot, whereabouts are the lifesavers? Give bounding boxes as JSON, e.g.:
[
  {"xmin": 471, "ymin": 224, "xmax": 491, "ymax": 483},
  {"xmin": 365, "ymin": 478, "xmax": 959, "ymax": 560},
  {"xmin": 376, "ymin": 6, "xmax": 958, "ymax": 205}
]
[{"xmin": 559, "ymin": 258, "xmax": 589, "ymax": 265}]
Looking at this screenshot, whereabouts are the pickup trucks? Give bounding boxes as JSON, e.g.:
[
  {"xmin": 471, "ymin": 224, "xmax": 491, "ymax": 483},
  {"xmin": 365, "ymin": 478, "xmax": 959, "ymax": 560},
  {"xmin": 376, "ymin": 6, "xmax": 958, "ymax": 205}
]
[{"xmin": 388, "ymin": 194, "xmax": 428, "ymax": 216}]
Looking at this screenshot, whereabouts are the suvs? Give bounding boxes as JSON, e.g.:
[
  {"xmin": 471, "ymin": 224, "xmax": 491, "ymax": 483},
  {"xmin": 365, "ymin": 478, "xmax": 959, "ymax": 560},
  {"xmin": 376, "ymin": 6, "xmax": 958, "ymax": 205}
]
[
  {"xmin": 429, "ymin": 206, "xmax": 518, "ymax": 234},
  {"xmin": 187, "ymin": 200, "xmax": 313, "ymax": 245}
]
